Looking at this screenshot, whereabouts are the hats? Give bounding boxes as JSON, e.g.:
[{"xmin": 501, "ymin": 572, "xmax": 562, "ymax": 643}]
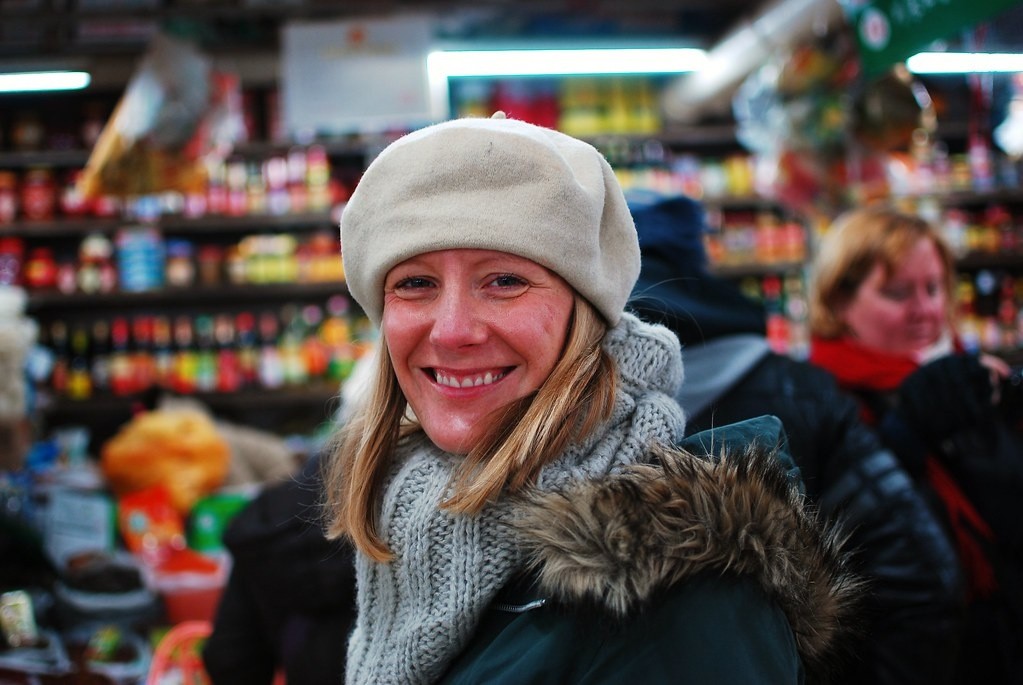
[{"xmin": 337, "ymin": 111, "xmax": 644, "ymax": 333}]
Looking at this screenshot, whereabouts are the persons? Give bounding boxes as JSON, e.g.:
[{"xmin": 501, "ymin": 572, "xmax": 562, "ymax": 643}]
[
  {"xmin": 624, "ymin": 186, "xmax": 969, "ymax": 685},
  {"xmin": 797, "ymin": 200, "xmax": 1022, "ymax": 685},
  {"xmin": 328, "ymin": 110, "xmax": 844, "ymax": 683}
]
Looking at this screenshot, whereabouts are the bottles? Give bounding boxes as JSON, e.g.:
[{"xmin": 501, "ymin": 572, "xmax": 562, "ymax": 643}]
[
  {"xmin": 491, "ymin": 67, "xmax": 1017, "ymax": 373},
  {"xmin": 0, "ymin": 115, "xmax": 438, "ymax": 412}
]
